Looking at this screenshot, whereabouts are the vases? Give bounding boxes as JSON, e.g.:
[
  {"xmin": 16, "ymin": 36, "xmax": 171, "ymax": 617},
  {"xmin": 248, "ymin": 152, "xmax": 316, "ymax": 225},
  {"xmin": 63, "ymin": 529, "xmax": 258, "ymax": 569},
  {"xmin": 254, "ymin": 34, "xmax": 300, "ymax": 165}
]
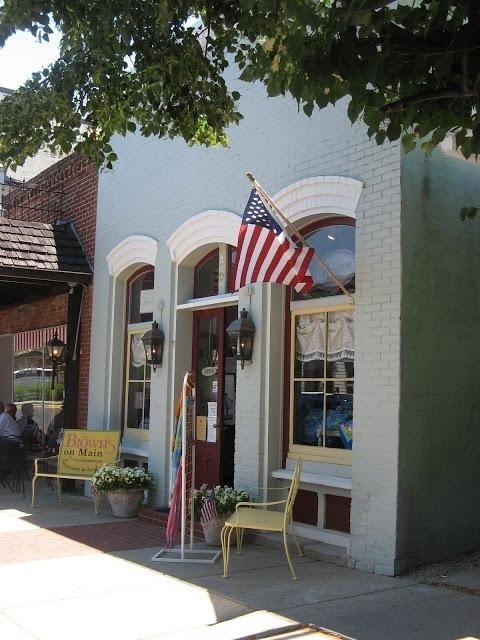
[
  {"xmin": 107, "ymin": 489, "xmax": 145, "ymax": 518},
  {"xmin": 200, "ymin": 514, "xmax": 242, "ymax": 547}
]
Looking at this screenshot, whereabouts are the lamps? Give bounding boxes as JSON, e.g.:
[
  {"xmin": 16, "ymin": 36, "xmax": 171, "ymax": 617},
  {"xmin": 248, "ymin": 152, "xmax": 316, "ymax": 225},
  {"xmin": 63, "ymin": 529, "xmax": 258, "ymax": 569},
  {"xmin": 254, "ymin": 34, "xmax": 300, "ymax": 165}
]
[
  {"xmin": 140, "ymin": 320, "xmax": 164, "ymax": 373},
  {"xmin": 43, "ymin": 331, "xmax": 77, "ymax": 390},
  {"xmin": 225, "ymin": 307, "xmax": 255, "ymax": 370}
]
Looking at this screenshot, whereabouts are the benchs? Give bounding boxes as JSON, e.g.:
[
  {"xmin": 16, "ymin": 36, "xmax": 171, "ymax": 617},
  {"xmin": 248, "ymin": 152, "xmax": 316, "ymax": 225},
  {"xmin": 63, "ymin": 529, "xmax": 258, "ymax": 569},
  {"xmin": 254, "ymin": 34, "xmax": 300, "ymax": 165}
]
[{"xmin": 30, "ymin": 428, "xmax": 121, "ymax": 514}]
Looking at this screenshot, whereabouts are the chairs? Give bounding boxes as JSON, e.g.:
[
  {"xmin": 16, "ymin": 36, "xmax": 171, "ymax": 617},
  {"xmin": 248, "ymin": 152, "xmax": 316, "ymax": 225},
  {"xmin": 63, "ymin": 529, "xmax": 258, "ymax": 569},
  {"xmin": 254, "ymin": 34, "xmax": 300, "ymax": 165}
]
[
  {"xmin": 220, "ymin": 456, "xmax": 305, "ymax": 581},
  {"xmin": 0, "ymin": 421, "xmax": 61, "ymax": 498}
]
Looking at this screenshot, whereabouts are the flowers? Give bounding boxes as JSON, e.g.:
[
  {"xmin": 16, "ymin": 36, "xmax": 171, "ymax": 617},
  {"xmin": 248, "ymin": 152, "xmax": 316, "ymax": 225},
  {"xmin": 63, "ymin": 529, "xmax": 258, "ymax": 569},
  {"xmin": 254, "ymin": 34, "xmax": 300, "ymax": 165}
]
[
  {"xmin": 188, "ymin": 482, "xmax": 253, "ymax": 520},
  {"xmin": 91, "ymin": 463, "xmax": 157, "ymax": 496}
]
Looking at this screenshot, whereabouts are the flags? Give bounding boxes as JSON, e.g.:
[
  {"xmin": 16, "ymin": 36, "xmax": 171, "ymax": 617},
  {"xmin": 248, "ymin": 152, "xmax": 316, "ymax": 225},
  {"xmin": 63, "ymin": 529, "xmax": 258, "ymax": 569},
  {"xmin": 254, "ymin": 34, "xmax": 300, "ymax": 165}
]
[
  {"xmin": 226, "ymin": 188, "xmax": 315, "ymax": 301},
  {"xmin": 199, "ymin": 488, "xmax": 219, "ymax": 525}
]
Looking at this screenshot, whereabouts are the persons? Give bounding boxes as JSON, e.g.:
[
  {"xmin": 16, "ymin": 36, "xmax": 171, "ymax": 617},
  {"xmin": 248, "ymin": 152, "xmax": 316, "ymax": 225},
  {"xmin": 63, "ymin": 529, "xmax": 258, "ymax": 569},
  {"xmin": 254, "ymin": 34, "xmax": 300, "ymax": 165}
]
[
  {"xmin": 16, "ymin": 403, "xmax": 39, "ymax": 440},
  {"xmin": 5, "ymin": 402, "xmax": 17, "ymax": 417},
  {"xmin": 0, "ymin": 404, "xmax": 23, "ymax": 447},
  {"xmin": 45, "ymin": 405, "xmax": 66, "ymax": 448}
]
